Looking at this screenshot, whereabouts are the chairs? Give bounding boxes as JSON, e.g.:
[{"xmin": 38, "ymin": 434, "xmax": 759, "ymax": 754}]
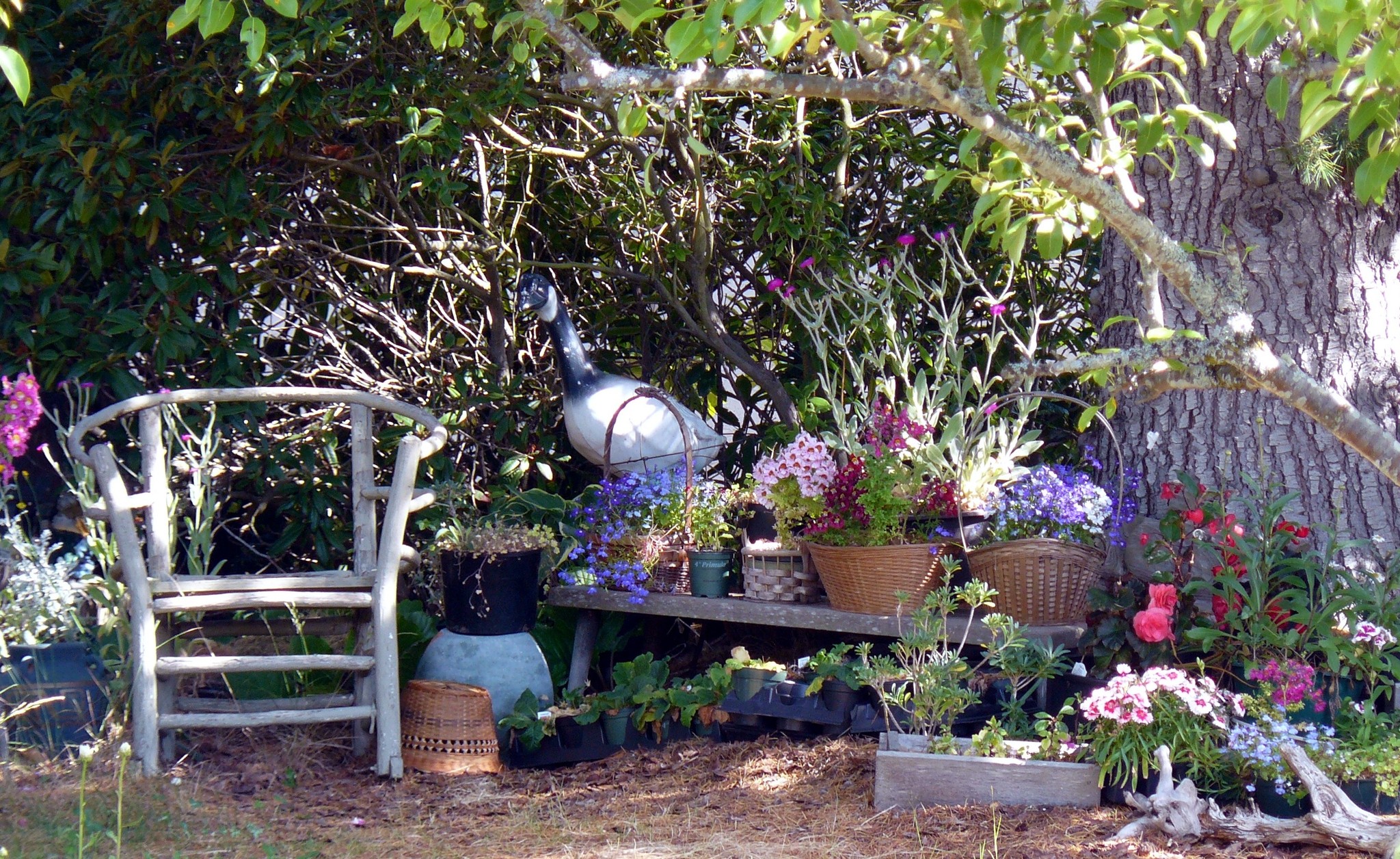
[{"xmin": 64, "ymin": 384, "xmax": 449, "ymax": 785}]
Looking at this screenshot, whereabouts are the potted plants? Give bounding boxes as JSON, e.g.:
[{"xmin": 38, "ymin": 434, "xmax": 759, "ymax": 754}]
[
  {"xmin": 812, "ymin": 646, "xmax": 864, "ymax": 710},
  {"xmin": 802, "ymin": 655, "xmax": 820, "ymax": 682},
  {"xmin": 870, "ymin": 652, "xmax": 909, "ymax": 707},
  {"xmin": 727, "ymin": 645, "xmax": 781, "ymax": 699},
  {"xmin": 431, "ymin": 503, "xmax": 729, "ymax": 755},
  {"xmin": 860, "ymin": 552, "xmax": 1102, "ymax": 815}
]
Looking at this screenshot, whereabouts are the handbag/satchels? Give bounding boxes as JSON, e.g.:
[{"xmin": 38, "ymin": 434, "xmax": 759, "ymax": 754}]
[
  {"xmin": 740, "ymin": 495, "xmax": 820, "ymax": 604},
  {"xmin": 954, "ymin": 391, "xmax": 1125, "ymax": 625}
]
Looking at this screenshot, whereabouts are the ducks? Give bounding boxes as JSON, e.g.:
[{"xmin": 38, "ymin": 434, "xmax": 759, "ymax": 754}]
[{"xmin": 516, "ymin": 274, "xmax": 727, "ymax": 481}]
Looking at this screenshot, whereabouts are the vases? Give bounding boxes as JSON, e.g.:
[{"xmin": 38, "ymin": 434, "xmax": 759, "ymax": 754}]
[
  {"xmin": 966, "ymin": 544, "xmax": 1100, "ymax": 629},
  {"xmin": 589, "ymin": 530, "xmax": 698, "ymax": 595},
  {"xmin": 562, "ymin": 220, "xmax": 1400, "ymax": 823},
  {"xmin": 1230, "ymin": 663, "xmax": 1377, "ymax": 735},
  {"xmin": 1257, "ymin": 770, "xmax": 1311, "ymax": 815},
  {"xmin": 0, "ymin": 643, "xmax": 111, "ymax": 752},
  {"xmin": 805, "ymin": 526, "xmax": 948, "ymax": 613},
  {"xmin": 1108, "ymin": 756, "xmax": 1150, "ymax": 809},
  {"xmin": 739, "ymin": 541, "xmax": 806, "ymax": 607},
  {"xmin": 1148, "ymin": 757, "xmax": 1201, "ymax": 804},
  {"xmin": 687, "ymin": 548, "xmax": 732, "ymax": 600},
  {"xmin": 1334, "ymin": 766, "xmax": 1376, "ymax": 813}
]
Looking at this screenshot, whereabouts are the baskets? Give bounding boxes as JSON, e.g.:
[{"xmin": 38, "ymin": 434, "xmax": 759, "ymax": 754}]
[
  {"xmin": 366, "ymin": 679, "xmax": 503, "ymax": 772},
  {"xmin": 587, "ymin": 387, "xmax": 729, "ymax": 593},
  {"xmin": 803, "ymin": 524, "xmax": 960, "ymax": 617}
]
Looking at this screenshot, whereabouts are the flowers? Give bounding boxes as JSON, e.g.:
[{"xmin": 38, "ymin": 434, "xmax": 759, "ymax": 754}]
[{"xmin": 0, "ymin": 368, "xmax": 118, "ymax": 754}]
